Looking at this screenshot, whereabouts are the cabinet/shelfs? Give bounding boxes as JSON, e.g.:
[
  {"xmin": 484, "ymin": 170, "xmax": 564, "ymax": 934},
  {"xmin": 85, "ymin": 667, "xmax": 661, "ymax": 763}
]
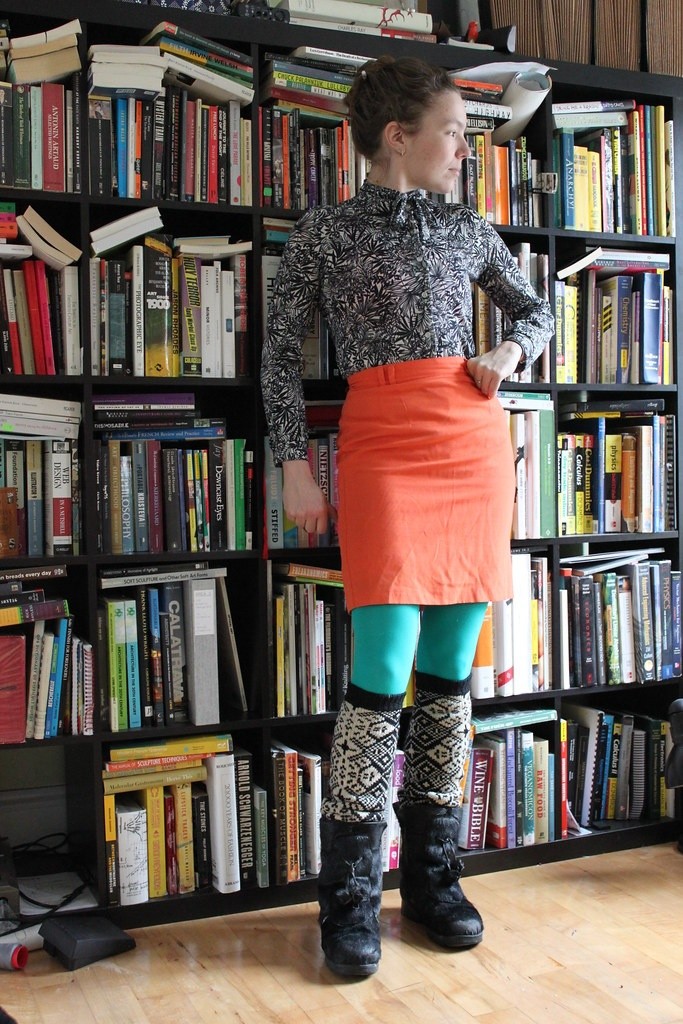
[{"xmin": 0, "ymin": 0, "xmax": 683, "ymax": 931}]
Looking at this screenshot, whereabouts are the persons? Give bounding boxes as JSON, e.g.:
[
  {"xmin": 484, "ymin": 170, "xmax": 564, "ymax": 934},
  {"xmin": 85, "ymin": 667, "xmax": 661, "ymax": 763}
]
[{"xmin": 261, "ymin": 55, "xmax": 556, "ymax": 976}]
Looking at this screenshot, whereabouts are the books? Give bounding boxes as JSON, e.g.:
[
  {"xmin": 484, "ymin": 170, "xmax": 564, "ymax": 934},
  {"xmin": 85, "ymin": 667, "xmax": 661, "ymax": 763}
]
[
  {"xmin": 103, "ymin": 702, "xmax": 683, "ymax": 906},
  {"xmin": 0, "ymin": 546, "xmax": 683, "ymax": 744},
  {"xmin": 0, "ymin": 204, "xmax": 677, "ymax": 388},
  {"xmin": 0, "ymin": 387, "xmax": 679, "ymax": 554},
  {"xmin": 0, "ymin": 0, "xmax": 675, "ymax": 237}
]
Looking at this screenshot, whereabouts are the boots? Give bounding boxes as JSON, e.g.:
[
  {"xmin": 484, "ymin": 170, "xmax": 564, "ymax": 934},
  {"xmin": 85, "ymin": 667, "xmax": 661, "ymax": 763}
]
[
  {"xmin": 392, "ymin": 801, "xmax": 484, "ymax": 948},
  {"xmin": 316, "ymin": 819, "xmax": 387, "ymax": 975}
]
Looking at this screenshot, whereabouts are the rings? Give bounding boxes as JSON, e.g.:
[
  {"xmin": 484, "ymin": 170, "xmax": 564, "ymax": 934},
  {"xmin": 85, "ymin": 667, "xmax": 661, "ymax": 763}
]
[{"xmin": 474, "ymin": 379, "xmax": 481, "ymax": 384}]
